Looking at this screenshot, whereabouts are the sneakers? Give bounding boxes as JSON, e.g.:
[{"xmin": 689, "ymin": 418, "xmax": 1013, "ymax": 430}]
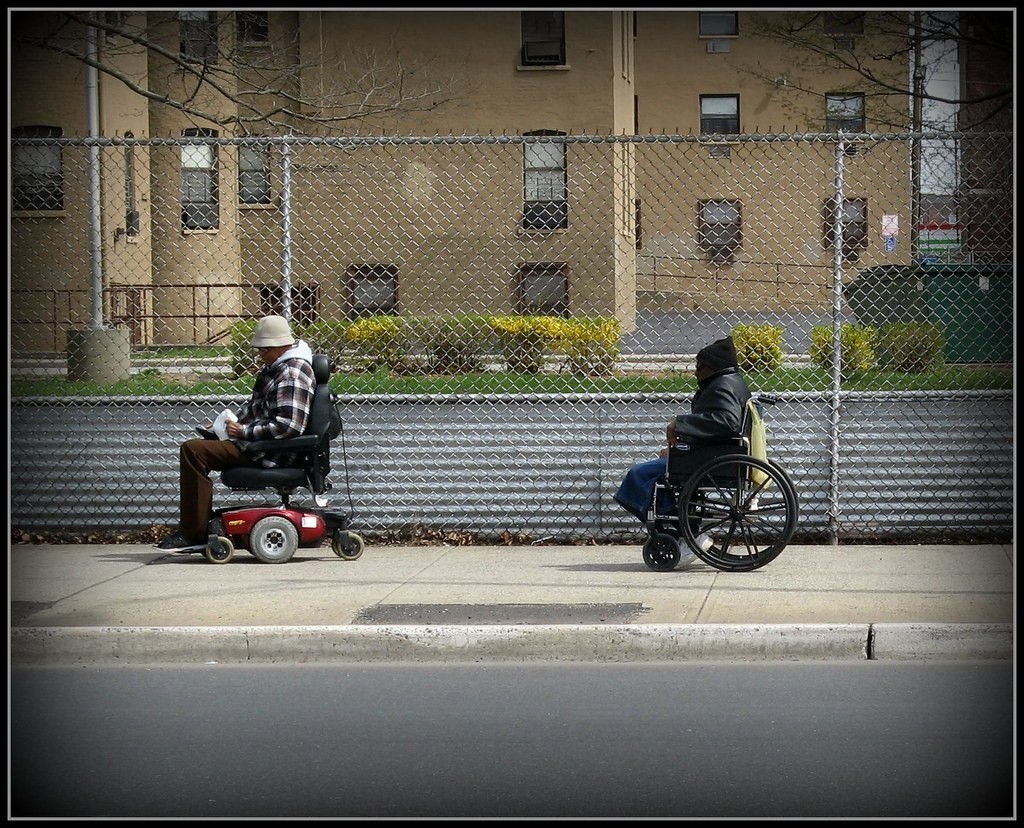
[
  {"xmin": 673, "ymin": 530, "xmax": 714, "ymax": 569},
  {"xmin": 151, "ymin": 530, "xmax": 208, "ymax": 552}
]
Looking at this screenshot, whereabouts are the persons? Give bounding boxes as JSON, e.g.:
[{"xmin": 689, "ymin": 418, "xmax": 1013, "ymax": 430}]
[
  {"xmin": 613, "ymin": 334, "xmax": 752, "ymax": 570},
  {"xmin": 151, "ymin": 315, "xmax": 317, "ymax": 554}
]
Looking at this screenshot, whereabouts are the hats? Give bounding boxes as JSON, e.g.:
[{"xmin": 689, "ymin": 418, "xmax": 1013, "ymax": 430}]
[
  {"xmin": 696, "ymin": 336, "xmax": 737, "ymax": 372},
  {"xmin": 250, "ymin": 315, "xmax": 295, "ymax": 347}
]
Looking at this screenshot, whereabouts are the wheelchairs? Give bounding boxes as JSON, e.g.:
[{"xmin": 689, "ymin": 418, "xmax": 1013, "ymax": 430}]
[
  {"xmin": 171, "ymin": 354, "xmax": 365, "ymax": 568},
  {"xmin": 641, "ymin": 397, "xmax": 801, "ymax": 574}
]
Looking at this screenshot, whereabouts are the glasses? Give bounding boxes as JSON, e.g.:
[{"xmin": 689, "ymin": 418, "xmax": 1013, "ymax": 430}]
[
  {"xmin": 696, "ymin": 361, "xmax": 708, "ymax": 373},
  {"xmin": 258, "ymin": 347, "xmax": 273, "ymax": 352}
]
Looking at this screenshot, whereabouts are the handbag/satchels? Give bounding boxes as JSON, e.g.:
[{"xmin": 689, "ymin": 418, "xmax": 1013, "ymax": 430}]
[{"xmin": 747, "ymin": 401, "xmax": 772, "ymax": 490}]
